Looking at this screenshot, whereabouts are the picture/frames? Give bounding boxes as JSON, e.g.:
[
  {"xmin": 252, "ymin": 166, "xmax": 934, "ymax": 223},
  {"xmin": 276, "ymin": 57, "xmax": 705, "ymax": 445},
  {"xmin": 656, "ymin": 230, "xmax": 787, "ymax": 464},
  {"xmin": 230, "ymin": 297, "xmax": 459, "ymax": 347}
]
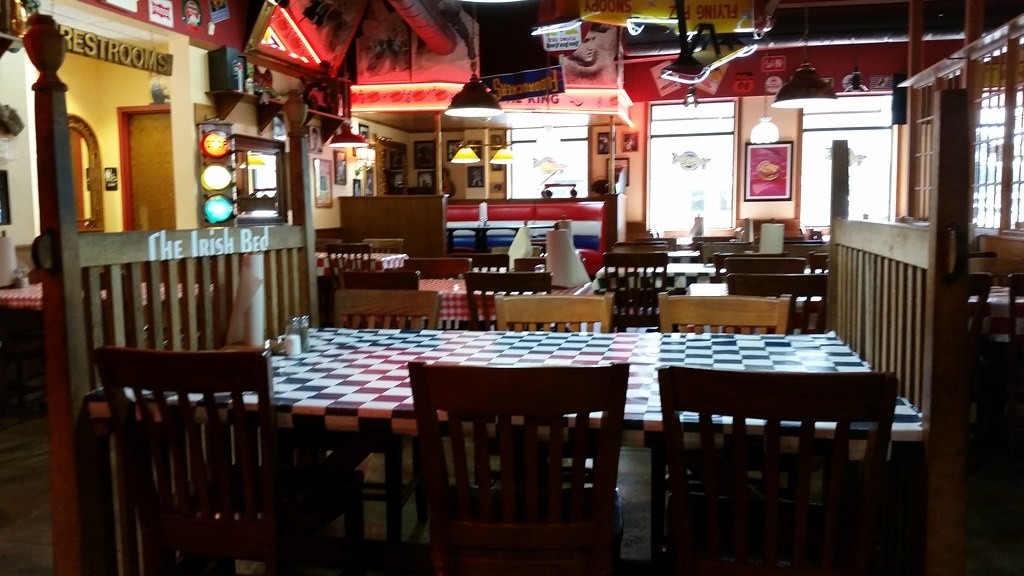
[
  {"xmin": 597, "ymin": 131, "xmax": 616, "ymax": 155},
  {"xmin": 385, "ymin": 148, "xmax": 403, "ymax": 190},
  {"xmin": 621, "ymin": 131, "xmax": 638, "ymax": 153},
  {"xmin": 606, "ymin": 158, "xmax": 630, "ymax": 187},
  {"xmin": 414, "ymin": 133, "xmax": 504, "ymax": 189},
  {"xmin": 744, "ymin": 140, "xmax": 793, "ymax": 202},
  {"xmin": 307, "ymin": 122, "xmax": 376, "ymax": 210}
]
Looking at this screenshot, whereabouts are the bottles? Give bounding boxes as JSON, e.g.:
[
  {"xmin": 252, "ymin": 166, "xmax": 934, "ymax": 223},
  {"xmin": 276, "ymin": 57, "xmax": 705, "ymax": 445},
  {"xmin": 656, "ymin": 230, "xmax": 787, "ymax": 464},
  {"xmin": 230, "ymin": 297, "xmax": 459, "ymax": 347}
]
[
  {"xmin": 535, "ymin": 265, "xmax": 545, "ymax": 273},
  {"xmin": 285, "ymin": 316, "xmax": 302, "ymax": 355},
  {"xmin": 299, "ymin": 314, "xmax": 310, "ymax": 351}
]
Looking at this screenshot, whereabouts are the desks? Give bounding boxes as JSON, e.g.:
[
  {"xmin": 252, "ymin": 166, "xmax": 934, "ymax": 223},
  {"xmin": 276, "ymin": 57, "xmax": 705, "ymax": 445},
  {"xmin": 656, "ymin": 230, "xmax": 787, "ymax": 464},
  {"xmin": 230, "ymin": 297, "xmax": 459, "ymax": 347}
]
[
  {"xmin": 84, "ymin": 327, "xmax": 927, "ymax": 576},
  {"xmin": 0, "ymin": 282, "xmax": 216, "ymax": 409},
  {"xmin": 595, "ymin": 263, "xmax": 829, "ymax": 292},
  {"xmin": 966, "ymin": 286, "xmax": 1024, "ymax": 342},
  {"xmin": 315, "ymin": 253, "xmax": 408, "ymax": 276},
  {"xmin": 660, "ymin": 249, "xmax": 703, "ymax": 263},
  {"xmin": 334, "ymin": 277, "xmax": 828, "ymax": 334}
]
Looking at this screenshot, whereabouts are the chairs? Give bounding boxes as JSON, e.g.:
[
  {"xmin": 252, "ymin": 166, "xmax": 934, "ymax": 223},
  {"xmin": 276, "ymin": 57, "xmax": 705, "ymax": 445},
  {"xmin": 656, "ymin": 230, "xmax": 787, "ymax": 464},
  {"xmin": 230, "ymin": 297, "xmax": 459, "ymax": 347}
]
[
  {"xmin": 95, "ymin": 240, "xmax": 922, "ymax": 576},
  {"xmin": 963, "ymin": 273, "xmax": 1024, "ymax": 470}
]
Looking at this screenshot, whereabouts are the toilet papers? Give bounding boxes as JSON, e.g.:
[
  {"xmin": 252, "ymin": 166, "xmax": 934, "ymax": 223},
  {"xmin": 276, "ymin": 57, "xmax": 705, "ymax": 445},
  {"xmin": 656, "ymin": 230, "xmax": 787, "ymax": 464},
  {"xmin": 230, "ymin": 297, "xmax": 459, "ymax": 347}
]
[
  {"xmin": 759, "ymin": 223, "xmax": 785, "ymax": 254},
  {"xmin": 225, "ymin": 252, "xmax": 265, "ymax": 346},
  {"xmin": 507, "ymin": 226, "xmax": 533, "ymax": 270},
  {"xmin": 689, "ymin": 216, "xmax": 704, "ymax": 237},
  {"xmin": 547, "ymin": 218, "xmax": 593, "ymax": 290},
  {"xmin": 0, "ymin": 236, "xmax": 18, "ymax": 287}
]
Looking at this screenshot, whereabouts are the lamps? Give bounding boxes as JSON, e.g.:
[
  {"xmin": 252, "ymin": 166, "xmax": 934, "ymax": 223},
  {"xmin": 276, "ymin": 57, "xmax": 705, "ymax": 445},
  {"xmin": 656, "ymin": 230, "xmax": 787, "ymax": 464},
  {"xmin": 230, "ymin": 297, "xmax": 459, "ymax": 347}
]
[
  {"xmin": 844, "ymin": 46, "xmax": 868, "ymax": 94},
  {"xmin": 451, "ymin": 143, "xmax": 516, "ymax": 164},
  {"xmin": 444, "ymin": 0, "xmax": 505, "ymax": 117},
  {"xmin": 750, "ymin": 93, "xmax": 780, "ymax": 144},
  {"xmin": 329, "ymin": 57, "xmax": 368, "ymax": 149},
  {"xmin": 239, "ymin": 152, "xmax": 268, "ymax": 170},
  {"xmin": 771, "ymin": 0, "xmax": 840, "ymax": 108}
]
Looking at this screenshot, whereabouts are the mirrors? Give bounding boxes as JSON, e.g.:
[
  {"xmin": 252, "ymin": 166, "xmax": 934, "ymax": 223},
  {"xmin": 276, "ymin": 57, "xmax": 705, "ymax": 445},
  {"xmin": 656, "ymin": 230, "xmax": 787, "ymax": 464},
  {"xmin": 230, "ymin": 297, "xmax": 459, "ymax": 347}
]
[
  {"xmin": 229, "ymin": 134, "xmax": 289, "ymax": 228},
  {"xmin": 375, "ymin": 137, "xmax": 408, "ymax": 195}
]
